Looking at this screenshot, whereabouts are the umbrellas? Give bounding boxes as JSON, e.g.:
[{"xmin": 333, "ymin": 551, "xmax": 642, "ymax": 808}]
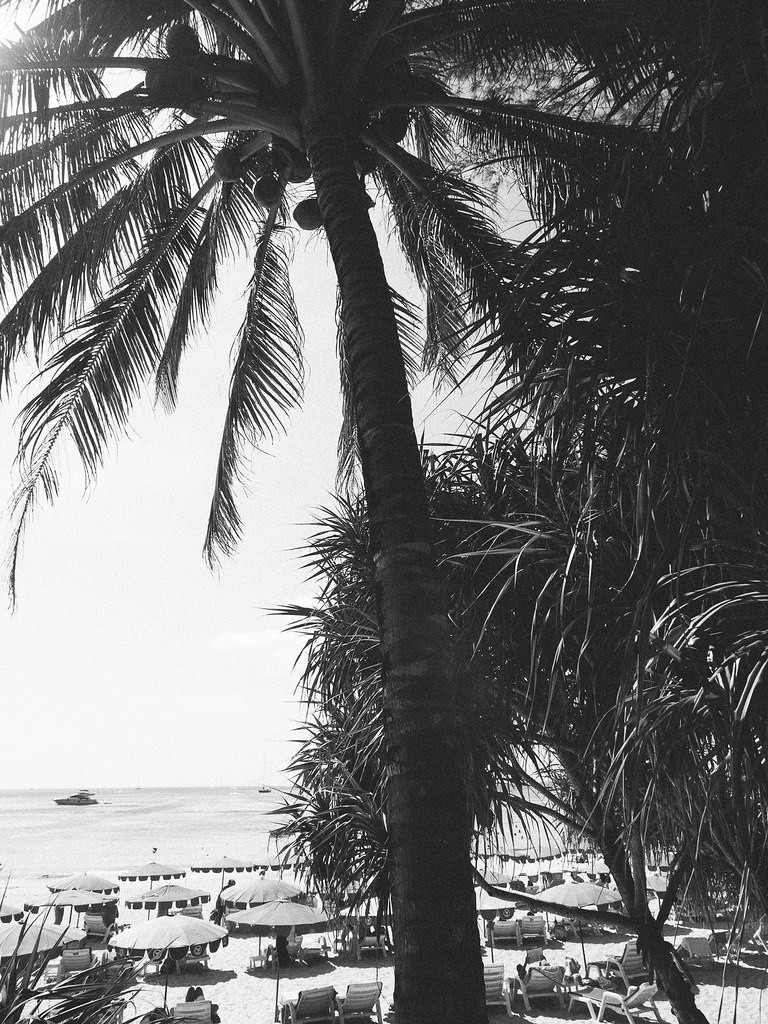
[
  {"xmin": 0, "ymin": 854, "xmax": 390, "ymax": 980},
  {"xmin": 469, "ymin": 831, "xmax": 672, "ymax": 938}
]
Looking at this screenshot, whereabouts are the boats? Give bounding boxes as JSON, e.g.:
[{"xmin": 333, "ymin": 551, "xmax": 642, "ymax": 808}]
[
  {"xmin": 53, "ymin": 789, "xmax": 100, "ymax": 806},
  {"xmin": 257, "ymin": 784, "xmax": 272, "ymax": 793}
]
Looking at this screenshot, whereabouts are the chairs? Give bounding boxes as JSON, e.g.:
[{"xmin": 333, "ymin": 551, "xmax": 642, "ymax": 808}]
[{"xmin": 61, "ymin": 879, "xmax": 730, "ymax": 1024}]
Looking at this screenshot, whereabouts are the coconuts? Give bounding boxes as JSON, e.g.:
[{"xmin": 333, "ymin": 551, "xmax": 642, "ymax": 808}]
[{"xmin": 146, "ymin": 25, "xmax": 407, "ymax": 231}]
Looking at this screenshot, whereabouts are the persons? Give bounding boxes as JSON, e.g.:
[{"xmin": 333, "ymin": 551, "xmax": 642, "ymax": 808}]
[
  {"xmin": 517, "ymin": 953, "xmax": 551, "ymax": 981},
  {"xmin": 102, "ymin": 902, "xmax": 119, "ymax": 926}
]
